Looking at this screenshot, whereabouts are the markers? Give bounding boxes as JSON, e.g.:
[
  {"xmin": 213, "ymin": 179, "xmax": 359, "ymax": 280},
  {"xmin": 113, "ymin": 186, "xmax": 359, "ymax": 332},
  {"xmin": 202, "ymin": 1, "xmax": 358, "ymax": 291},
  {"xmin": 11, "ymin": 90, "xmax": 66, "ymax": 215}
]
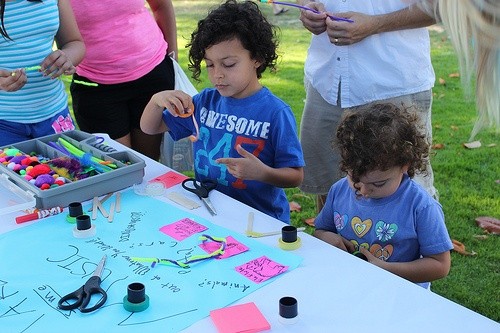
[{"xmin": 15, "ymin": 207, "xmax": 63, "ymax": 224}]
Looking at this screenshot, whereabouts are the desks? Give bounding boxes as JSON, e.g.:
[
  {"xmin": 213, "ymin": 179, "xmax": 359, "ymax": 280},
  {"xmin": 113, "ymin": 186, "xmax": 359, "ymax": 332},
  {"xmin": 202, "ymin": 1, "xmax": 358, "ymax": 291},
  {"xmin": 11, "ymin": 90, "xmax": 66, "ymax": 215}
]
[{"xmin": 0, "ymin": 144, "xmax": 500, "ymax": 333}]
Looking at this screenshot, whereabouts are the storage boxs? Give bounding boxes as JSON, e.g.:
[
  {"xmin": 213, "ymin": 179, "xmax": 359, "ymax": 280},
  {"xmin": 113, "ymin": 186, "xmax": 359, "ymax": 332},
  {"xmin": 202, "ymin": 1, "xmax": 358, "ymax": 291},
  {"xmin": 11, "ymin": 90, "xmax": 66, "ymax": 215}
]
[
  {"xmin": 0, "ymin": 129, "xmax": 146, "ymax": 211},
  {"xmin": 0, "ymin": 176, "xmax": 37, "ymax": 225}
]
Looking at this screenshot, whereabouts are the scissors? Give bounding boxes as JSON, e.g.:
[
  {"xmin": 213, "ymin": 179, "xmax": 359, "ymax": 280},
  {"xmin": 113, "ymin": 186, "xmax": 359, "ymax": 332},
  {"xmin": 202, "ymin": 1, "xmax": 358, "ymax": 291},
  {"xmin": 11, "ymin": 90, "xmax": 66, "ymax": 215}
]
[
  {"xmin": 57, "ymin": 254, "xmax": 108, "ymax": 314},
  {"xmin": 181, "ymin": 177, "xmax": 219, "ymax": 216}
]
